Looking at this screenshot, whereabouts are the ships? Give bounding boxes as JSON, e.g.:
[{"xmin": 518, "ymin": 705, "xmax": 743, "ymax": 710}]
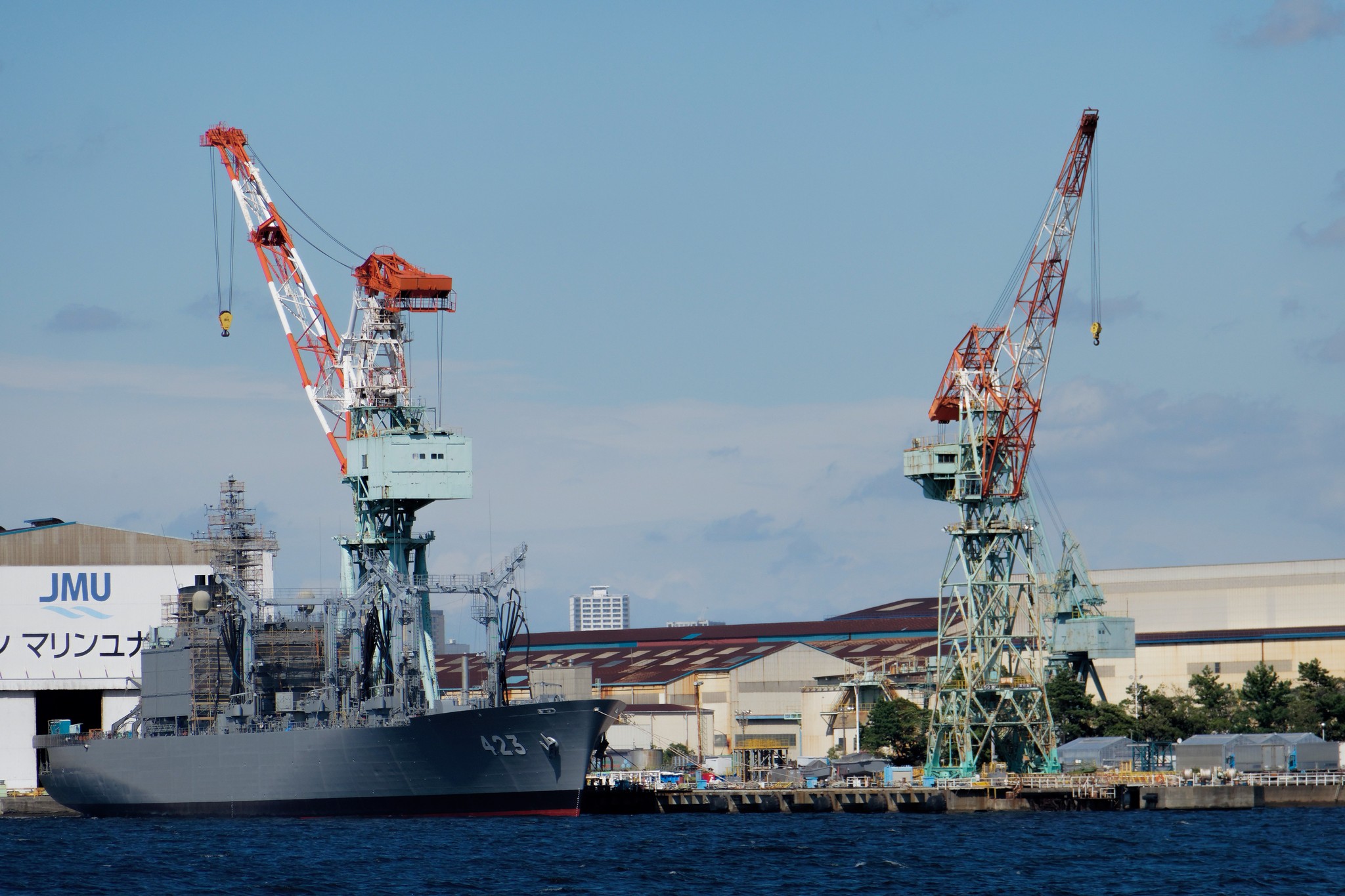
[{"xmin": 34, "ymin": 474, "xmax": 628, "ymax": 819}]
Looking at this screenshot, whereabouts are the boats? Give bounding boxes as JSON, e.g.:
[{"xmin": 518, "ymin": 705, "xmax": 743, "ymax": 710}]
[
  {"xmin": 799, "ymin": 760, "xmax": 832, "ymax": 780},
  {"xmin": 830, "ymin": 753, "xmax": 891, "ymax": 778}
]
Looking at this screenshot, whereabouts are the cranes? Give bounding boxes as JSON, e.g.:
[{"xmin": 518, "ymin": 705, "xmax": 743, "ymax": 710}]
[
  {"xmin": 902, "ymin": 106, "xmax": 1102, "ymax": 789},
  {"xmin": 198, "ymin": 119, "xmax": 475, "ymax": 711}
]
